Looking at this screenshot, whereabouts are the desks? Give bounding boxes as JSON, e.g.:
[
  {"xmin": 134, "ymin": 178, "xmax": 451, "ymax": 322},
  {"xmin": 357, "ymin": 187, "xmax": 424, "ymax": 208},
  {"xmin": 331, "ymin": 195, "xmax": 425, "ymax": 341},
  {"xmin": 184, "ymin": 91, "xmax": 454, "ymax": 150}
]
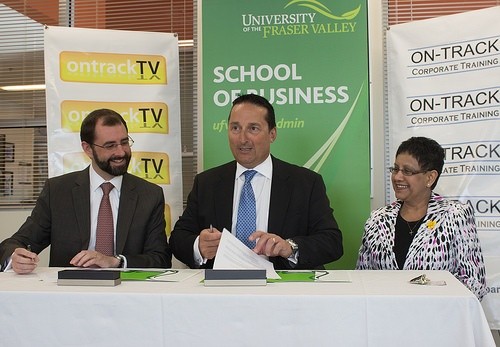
[{"xmin": 0, "ymin": 267, "xmax": 497, "ymax": 347}]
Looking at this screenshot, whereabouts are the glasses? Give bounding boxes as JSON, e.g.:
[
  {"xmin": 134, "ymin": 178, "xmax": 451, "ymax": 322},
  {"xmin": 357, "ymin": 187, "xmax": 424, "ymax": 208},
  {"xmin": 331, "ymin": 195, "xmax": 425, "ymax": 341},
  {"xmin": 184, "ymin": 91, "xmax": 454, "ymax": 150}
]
[
  {"xmin": 86, "ymin": 134, "xmax": 134, "ymax": 151},
  {"xmin": 389, "ymin": 167, "xmax": 427, "ymax": 176}
]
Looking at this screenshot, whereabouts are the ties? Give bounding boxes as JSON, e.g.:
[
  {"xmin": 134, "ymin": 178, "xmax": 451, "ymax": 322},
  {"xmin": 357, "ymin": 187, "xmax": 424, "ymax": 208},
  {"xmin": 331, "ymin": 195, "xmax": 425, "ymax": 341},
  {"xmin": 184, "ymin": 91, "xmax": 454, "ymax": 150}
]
[
  {"xmin": 94, "ymin": 183, "xmax": 115, "ymax": 257},
  {"xmin": 236, "ymin": 170, "xmax": 258, "ymax": 251}
]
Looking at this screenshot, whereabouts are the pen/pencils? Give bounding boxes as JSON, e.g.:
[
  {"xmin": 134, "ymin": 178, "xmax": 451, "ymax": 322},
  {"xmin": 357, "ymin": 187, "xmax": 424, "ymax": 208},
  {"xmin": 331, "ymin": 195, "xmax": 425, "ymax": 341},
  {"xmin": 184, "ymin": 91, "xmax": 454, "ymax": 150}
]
[
  {"xmin": 210, "ymin": 225, "xmax": 213, "ymax": 233},
  {"xmin": 27, "ymin": 245, "xmax": 35, "ymax": 263}
]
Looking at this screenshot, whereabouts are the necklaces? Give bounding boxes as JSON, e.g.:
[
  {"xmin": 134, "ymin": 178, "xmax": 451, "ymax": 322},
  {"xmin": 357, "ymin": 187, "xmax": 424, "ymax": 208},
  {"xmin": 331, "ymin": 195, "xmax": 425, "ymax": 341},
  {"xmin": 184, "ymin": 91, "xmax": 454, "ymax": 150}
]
[{"xmin": 401, "ymin": 203, "xmax": 427, "ymax": 236}]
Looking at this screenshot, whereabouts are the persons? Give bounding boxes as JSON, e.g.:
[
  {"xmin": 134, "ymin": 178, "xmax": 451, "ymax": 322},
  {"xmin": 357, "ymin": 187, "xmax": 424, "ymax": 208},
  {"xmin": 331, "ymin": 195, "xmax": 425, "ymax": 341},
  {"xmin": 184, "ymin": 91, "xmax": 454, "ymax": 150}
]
[
  {"xmin": 0, "ymin": 109, "xmax": 172, "ymax": 275},
  {"xmin": 355, "ymin": 136, "xmax": 487, "ymax": 302},
  {"xmin": 168, "ymin": 94, "xmax": 344, "ymax": 270}
]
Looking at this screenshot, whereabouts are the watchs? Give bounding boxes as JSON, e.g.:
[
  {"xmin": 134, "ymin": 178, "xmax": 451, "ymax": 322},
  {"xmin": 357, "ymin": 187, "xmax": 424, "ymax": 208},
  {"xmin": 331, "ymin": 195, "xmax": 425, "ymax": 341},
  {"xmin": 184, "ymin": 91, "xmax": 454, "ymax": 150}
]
[
  {"xmin": 114, "ymin": 254, "xmax": 125, "ymax": 268},
  {"xmin": 286, "ymin": 237, "xmax": 298, "ymax": 258}
]
